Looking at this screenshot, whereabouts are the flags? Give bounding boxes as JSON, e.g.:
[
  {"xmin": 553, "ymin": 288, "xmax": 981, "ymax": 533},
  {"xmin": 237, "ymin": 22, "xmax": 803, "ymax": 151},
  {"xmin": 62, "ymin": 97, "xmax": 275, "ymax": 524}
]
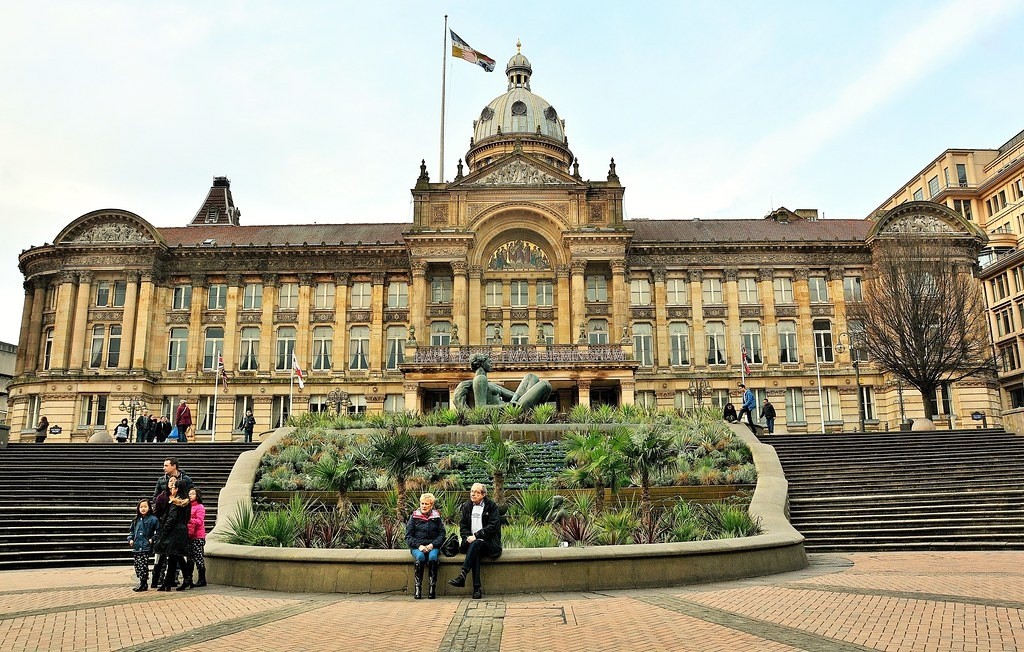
[
  {"xmin": 450, "ymin": 29, "xmax": 496, "ymax": 72},
  {"xmin": 294, "ymin": 354, "xmax": 304, "ymax": 389},
  {"xmin": 742, "ymin": 347, "xmax": 750, "ymax": 375},
  {"xmin": 219, "ymin": 353, "xmax": 228, "ymax": 391}
]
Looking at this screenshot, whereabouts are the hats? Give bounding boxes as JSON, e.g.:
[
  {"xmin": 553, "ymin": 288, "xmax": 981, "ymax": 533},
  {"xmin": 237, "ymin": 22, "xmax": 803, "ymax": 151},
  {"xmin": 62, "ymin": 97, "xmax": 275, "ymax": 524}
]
[{"xmin": 180, "ymin": 400, "xmax": 187, "ymax": 404}]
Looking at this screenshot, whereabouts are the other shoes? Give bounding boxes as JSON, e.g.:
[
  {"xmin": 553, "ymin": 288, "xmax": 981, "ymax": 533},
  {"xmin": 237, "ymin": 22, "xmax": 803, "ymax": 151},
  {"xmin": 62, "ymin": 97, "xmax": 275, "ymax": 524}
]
[
  {"xmin": 448, "ymin": 578, "xmax": 466, "ymax": 587},
  {"xmin": 472, "ymin": 588, "xmax": 482, "ymax": 599},
  {"xmin": 732, "ymin": 420, "xmax": 740, "ymax": 424}
]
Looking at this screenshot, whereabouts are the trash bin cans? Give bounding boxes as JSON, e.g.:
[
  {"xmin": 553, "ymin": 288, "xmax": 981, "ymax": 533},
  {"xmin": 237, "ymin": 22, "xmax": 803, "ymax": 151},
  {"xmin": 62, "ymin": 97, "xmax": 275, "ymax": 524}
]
[
  {"xmin": 0, "ymin": 424, "xmax": 11, "ymax": 449},
  {"xmin": 899, "ymin": 423, "xmax": 910, "ymax": 431}
]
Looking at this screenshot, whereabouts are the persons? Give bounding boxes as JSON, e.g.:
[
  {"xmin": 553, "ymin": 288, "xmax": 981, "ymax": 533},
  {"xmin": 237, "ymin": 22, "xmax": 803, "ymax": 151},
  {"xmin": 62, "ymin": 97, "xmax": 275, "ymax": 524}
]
[
  {"xmin": 35, "ymin": 417, "xmax": 49, "ymax": 443},
  {"xmin": 732, "ymin": 384, "xmax": 758, "ymax": 435},
  {"xmin": 447, "ymin": 481, "xmax": 502, "ymax": 599},
  {"xmin": 471, "ymin": 351, "xmax": 552, "ymax": 412},
  {"xmin": 127, "ymin": 457, "xmax": 207, "ymax": 591},
  {"xmin": 545, "ymin": 495, "xmax": 571, "ymax": 524},
  {"xmin": 404, "ymin": 493, "xmax": 446, "ymax": 598},
  {"xmin": 176, "ymin": 399, "xmax": 192, "ymax": 442},
  {"xmin": 114, "ymin": 417, "xmax": 130, "ymax": 442},
  {"xmin": 135, "ymin": 409, "xmax": 172, "ymax": 442},
  {"xmin": 723, "ymin": 403, "xmax": 737, "ymax": 423},
  {"xmin": 240, "ymin": 408, "xmax": 256, "ymax": 442},
  {"xmin": 758, "ymin": 399, "xmax": 776, "ymax": 434}
]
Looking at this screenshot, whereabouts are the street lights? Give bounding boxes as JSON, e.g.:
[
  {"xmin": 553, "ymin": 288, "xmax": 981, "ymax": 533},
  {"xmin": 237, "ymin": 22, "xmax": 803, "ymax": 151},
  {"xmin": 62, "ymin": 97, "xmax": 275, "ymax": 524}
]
[
  {"xmin": 835, "ymin": 332, "xmax": 871, "ymax": 432},
  {"xmin": 119, "ymin": 395, "xmax": 148, "ymax": 443},
  {"xmin": 325, "ymin": 391, "xmax": 349, "ymax": 420},
  {"xmin": 687, "ymin": 378, "xmax": 713, "ymax": 413}
]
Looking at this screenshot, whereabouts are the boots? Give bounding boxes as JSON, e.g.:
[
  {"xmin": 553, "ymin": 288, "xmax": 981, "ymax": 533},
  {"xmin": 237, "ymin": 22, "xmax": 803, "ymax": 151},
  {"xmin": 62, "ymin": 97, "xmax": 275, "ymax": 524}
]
[
  {"xmin": 133, "ymin": 579, "xmax": 148, "ymax": 592},
  {"xmin": 158, "ymin": 569, "xmax": 175, "ymax": 592},
  {"xmin": 414, "ymin": 561, "xmax": 424, "ymax": 598},
  {"xmin": 427, "ymin": 560, "xmax": 437, "ymax": 599},
  {"xmin": 176, "ymin": 567, "xmax": 193, "ymax": 590},
  {"xmin": 150, "ymin": 564, "xmax": 161, "ymax": 589},
  {"xmin": 193, "ymin": 568, "xmax": 207, "ymax": 587}
]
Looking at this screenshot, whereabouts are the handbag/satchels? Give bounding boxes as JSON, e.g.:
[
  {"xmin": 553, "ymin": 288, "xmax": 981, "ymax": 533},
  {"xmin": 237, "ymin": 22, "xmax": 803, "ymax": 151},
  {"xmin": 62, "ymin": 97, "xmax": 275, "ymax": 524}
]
[
  {"xmin": 441, "ymin": 534, "xmax": 459, "ymax": 557},
  {"xmin": 186, "ymin": 427, "xmax": 193, "ymax": 437},
  {"xmin": 166, "ymin": 425, "xmax": 179, "ymax": 439}
]
